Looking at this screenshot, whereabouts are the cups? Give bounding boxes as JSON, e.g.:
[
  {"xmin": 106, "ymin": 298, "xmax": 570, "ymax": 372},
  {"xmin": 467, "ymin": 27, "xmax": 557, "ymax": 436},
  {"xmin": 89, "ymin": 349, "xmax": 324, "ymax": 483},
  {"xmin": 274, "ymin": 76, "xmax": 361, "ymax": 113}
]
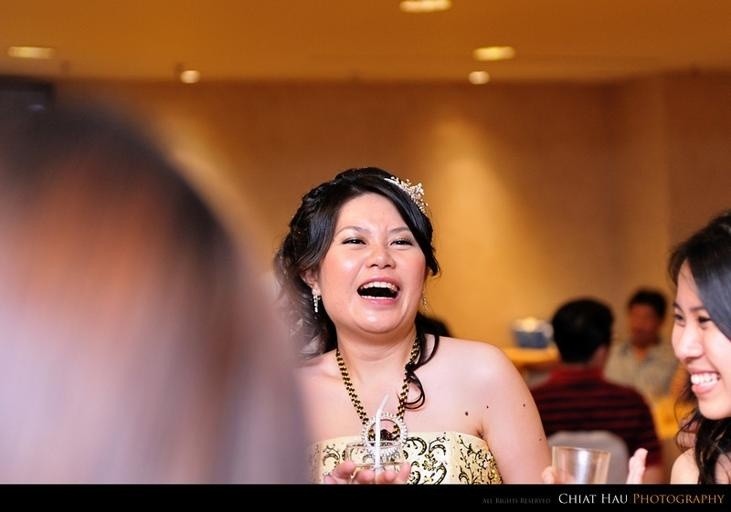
[{"xmin": 551, "ymin": 445, "xmax": 612, "ymax": 484}]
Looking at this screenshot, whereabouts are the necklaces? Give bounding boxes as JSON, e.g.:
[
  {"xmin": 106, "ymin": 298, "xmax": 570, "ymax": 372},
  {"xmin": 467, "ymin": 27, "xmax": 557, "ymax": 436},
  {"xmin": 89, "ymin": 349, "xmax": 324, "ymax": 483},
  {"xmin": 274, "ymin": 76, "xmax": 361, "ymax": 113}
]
[{"xmin": 334, "ymin": 334, "xmax": 422, "ymax": 458}]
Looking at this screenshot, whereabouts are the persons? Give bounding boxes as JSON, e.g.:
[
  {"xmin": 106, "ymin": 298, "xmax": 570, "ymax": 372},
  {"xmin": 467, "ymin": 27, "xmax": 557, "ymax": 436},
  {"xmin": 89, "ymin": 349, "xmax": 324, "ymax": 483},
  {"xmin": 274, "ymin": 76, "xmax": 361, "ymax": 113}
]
[
  {"xmin": 526, "ymin": 294, "xmax": 666, "ymax": 484},
  {"xmin": 603, "ymin": 283, "xmax": 684, "ymax": 409},
  {"xmin": 1, "ymin": 67, "xmax": 316, "ymax": 485},
  {"xmin": 626, "ymin": 207, "xmax": 731, "ymax": 485},
  {"xmin": 268, "ymin": 165, "xmax": 586, "ymax": 485}
]
